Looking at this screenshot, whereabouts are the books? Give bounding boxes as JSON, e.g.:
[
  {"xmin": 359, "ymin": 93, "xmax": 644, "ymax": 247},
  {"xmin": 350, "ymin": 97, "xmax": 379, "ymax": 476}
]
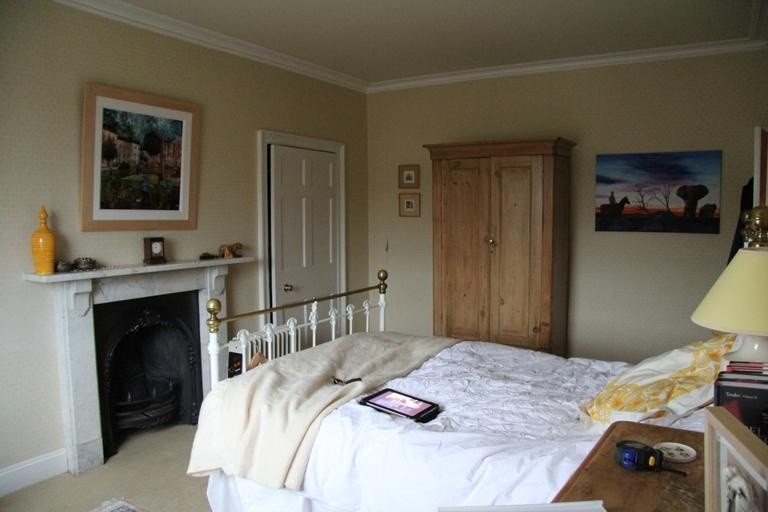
[{"xmin": 712, "ymin": 360, "xmax": 767, "ymax": 449}]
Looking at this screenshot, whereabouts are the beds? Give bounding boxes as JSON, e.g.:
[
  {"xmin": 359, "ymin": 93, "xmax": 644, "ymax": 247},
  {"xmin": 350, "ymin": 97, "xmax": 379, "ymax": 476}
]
[{"xmin": 208, "ymin": 206, "xmax": 768, "ymax": 512}]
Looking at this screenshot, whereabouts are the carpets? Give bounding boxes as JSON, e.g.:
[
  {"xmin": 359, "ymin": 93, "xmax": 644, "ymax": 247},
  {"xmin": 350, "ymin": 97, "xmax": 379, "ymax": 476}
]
[{"xmin": 90, "ymin": 497, "xmax": 141, "ymax": 512}]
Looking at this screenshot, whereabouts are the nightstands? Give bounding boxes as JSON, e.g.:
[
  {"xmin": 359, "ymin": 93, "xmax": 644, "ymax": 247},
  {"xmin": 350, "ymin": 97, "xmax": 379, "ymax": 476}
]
[{"xmin": 552, "ymin": 421, "xmax": 704, "ymax": 512}]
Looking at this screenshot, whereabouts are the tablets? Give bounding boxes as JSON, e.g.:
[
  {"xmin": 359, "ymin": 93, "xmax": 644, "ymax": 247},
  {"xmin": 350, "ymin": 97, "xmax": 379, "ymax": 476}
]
[{"xmin": 360, "ymin": 389, "xmax": 438, "ymax": 422}]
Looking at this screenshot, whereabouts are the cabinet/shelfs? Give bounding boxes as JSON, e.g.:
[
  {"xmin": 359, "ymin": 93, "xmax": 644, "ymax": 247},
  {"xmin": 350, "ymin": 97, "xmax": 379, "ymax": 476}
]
[{"xmin": 422, "ymin": 135, "xmax": 577, "ymax": 358}]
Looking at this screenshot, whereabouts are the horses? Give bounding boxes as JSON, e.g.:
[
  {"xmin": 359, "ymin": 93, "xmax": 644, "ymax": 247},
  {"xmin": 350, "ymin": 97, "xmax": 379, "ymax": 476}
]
[{"xmin": 600, "ymin": 196, "xmax": 631, "ymax": 222}]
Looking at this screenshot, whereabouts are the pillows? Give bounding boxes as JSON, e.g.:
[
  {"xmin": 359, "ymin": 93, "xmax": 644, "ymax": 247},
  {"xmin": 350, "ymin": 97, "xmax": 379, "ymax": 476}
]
[{"xmin": 584, "ymin": 333, "xmax": 743, "ymax": 427}]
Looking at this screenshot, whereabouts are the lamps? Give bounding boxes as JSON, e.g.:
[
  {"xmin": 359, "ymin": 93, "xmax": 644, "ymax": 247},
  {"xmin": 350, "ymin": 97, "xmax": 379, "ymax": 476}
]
[{"xmin": 690, "ymin": 250, "xmax": 768, "ymax": 363}]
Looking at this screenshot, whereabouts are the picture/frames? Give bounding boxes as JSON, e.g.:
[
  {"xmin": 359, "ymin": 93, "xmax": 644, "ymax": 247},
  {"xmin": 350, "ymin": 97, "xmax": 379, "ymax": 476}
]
[
  {"xmin": 704, "ymin": 406, "xmax": 768, "ymax": 512},
  {"xmin": 399, "ymin": 165, "xmax": 420, "ymax": 188},
  {"xmin": 82, "ymin": 81, "xmax": 201, "ymax": 231},
  {"xmin": 399, "ymin": 193, "xmax": 420, "ymax": 217}
]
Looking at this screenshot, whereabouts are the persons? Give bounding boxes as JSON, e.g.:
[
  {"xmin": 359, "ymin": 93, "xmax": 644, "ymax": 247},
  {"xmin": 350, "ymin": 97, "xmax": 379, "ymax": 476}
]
[{"xmin": 608, "ymin": 190, "xmax": 617, "ymax": 204}]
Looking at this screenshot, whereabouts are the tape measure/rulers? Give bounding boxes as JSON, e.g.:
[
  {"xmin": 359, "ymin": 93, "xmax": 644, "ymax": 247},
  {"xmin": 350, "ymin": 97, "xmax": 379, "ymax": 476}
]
[{"xmin": 616, "ymin": 440, "xmax": 662, "ymax": 471}]
